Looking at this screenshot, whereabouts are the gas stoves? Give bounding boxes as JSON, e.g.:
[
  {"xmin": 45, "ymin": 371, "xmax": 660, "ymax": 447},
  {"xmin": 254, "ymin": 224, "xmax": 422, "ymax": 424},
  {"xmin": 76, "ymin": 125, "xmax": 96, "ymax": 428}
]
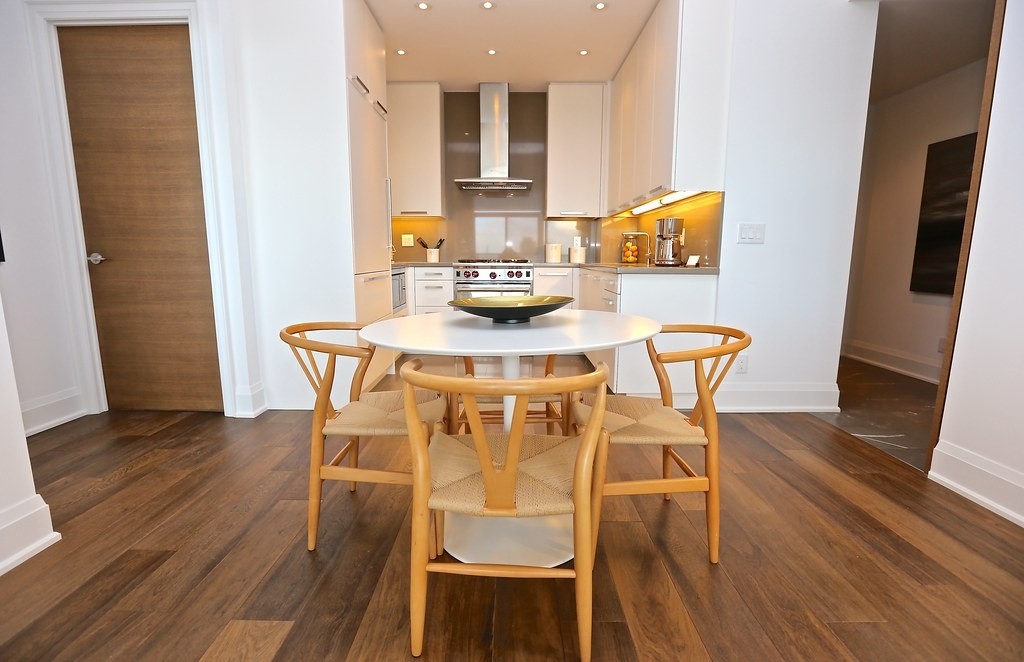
[{"xmin": 453, "ymin": 258, "xmax": 533, "ymax": 284}]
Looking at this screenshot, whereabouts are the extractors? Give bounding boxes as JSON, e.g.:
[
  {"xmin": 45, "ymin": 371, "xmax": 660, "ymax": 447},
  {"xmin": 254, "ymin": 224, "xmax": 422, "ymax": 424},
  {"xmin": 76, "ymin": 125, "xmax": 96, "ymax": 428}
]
[{"xmin": 454, "ymin": 82, "xmax": 534, "ymax": 191}]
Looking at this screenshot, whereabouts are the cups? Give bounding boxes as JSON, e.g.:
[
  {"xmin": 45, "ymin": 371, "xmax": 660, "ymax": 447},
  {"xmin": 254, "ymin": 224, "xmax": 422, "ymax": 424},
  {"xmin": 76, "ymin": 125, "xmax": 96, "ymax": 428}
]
[
  {"xmin": 426, "ymin": 249, "xmax": 440, "ymax": 264},
  {"xmin": 569, "ymin": 246, "xmax": 587, "ymax": 265},
  {"xmin": 545, "ymin": 243, "xmax": 562, "ymax": 264}
]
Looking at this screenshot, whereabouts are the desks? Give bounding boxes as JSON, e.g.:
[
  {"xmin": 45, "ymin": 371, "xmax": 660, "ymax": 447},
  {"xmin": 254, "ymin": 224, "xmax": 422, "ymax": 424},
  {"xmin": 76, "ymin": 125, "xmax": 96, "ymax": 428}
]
[{"xmin": 359, "ymin": 309, "xmax": 662, "ymax": 569}]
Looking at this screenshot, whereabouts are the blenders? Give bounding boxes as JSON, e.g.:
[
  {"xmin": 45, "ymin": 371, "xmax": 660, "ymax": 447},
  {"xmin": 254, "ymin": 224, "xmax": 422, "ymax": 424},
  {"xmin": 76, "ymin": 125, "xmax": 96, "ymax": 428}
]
[{"xmin": 653, "ymin": 217, "xmax": 685, "ymax": 267}]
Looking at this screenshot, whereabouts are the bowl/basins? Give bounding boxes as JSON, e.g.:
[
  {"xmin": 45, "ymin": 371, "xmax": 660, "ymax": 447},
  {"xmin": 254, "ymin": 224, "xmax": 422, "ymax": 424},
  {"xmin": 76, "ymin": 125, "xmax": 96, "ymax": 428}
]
[{"xmin": 447, "ymin": 295, "xmax": 575, "ymax": 325}]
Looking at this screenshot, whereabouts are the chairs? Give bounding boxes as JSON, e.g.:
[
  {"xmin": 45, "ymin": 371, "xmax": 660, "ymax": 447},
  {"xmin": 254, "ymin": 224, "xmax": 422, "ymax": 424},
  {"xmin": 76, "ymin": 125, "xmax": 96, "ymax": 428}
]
[
  {"xmin": 279, "ymin": 322, "xmax": 450, "ymax": 560},
  {"xmin": 399, "ymin": 358, "xmax": 610, "ymax": 662},
  {"xmin": 571, "ymin": 325, "xmax": 752, "ymax": 571}
]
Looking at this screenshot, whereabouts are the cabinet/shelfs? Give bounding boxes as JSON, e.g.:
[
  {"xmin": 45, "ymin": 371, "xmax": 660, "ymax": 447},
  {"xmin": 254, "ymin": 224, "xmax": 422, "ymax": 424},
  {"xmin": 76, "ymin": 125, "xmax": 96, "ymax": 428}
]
[
  {"xmin": 392, "ymin": 266, "xmax": 719, "ymax": 410},
  {"xmin": 386, "ymin": 82, "xmax": 445, "ymax": 221},
  {"xmin": 546, "ymin": 1, "xmax": 731, "ymax": 221}
]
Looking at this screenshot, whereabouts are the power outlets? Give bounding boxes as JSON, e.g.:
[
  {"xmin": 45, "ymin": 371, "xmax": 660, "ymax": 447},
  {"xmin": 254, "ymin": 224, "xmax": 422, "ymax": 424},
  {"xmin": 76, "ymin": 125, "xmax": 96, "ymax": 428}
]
[{"xmin": 574, "ymin": 237, "xmax": 581, "ymax": 247}]
